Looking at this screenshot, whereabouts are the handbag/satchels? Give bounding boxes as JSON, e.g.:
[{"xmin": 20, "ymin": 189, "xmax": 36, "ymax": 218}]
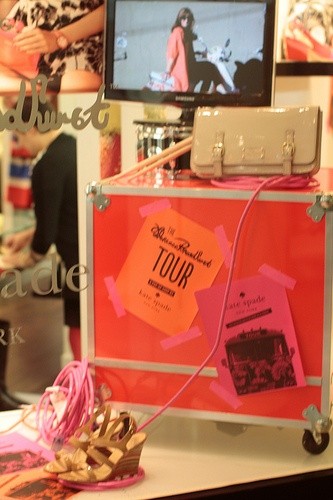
[
  {"xmin": 188, "ymin": 105, "xmax": 321, "ymax": 178},
  {"xmin": 0, "ymin": 20, "xmax": 40, "ymax": 80}
]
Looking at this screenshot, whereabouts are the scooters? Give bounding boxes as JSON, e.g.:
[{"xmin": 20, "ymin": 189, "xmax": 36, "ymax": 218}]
[{"xmin": 144, "ymin": 36, "xmax": 237, "ymax": 94}]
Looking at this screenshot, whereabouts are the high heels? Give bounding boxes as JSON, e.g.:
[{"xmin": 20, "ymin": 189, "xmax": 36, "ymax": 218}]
[{"xmin": 42, "ymin": 404, "xmax": 147, "ymax": 483}]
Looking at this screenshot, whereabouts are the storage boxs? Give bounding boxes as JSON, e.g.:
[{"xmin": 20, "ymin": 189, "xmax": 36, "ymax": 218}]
[{"xmin": 80, "ymin": 136, "xmax": 333, "ymax": 432}]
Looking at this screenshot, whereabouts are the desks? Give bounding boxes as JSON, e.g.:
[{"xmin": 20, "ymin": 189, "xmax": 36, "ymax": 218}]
[{"xmin": 1, "ymin": 408, "xmax": 333, "ymax": 500}]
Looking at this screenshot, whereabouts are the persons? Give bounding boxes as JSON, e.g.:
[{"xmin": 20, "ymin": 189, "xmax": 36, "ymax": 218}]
[
  {"xmin": 164, "ymin": 7, "xmax": 232, "ymax": 94},
  {"xmin": 9, "ymin": 96, "xmax": 81, "ymax": 363}
]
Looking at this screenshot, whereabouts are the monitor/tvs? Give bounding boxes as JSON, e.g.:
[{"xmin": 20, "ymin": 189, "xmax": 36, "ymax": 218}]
[{"xmin": 102, "ymin": 0, "xmax": 277, "ymax": 110}]
